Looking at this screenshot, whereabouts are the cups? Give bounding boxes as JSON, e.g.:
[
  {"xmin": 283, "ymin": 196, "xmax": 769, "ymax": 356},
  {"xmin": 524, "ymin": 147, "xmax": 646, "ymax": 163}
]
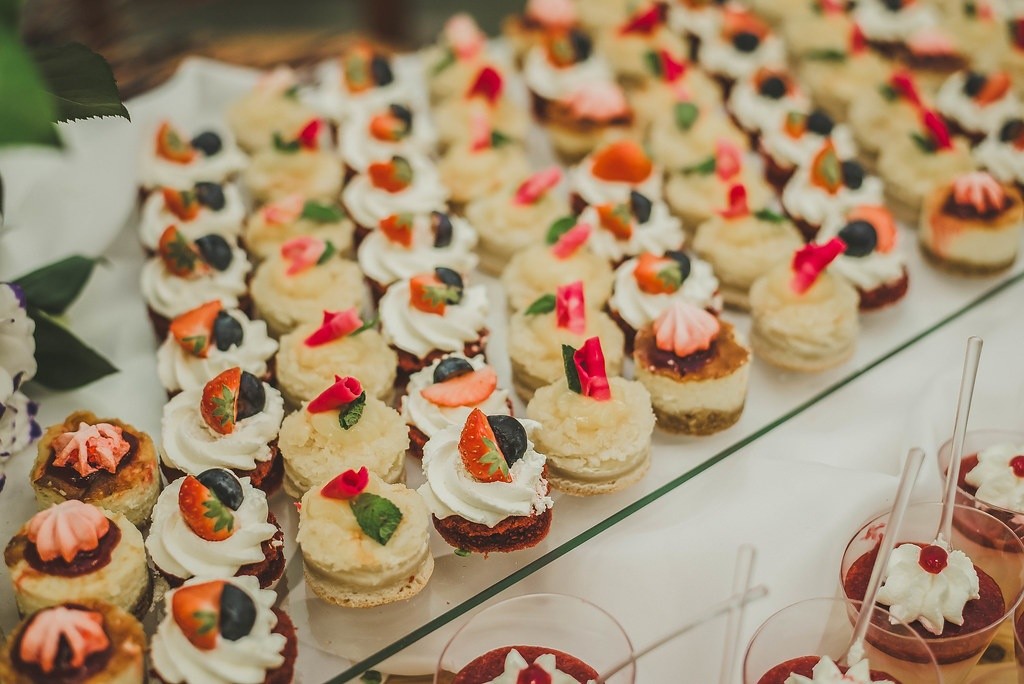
[
  {"xmin": 742, "ymin": 597, "xmax": 943, "ymax": 684},
  {"xmin": 433, "ymin": 594, "xmax": 637, "ymax": 684},
  {"xmin": 935, "ymin": 429, "xmax": 1024, "ymax": 598},
  {"xmin": 839, "ymin": 503, "xmax": 1024, "ymax": 683}
]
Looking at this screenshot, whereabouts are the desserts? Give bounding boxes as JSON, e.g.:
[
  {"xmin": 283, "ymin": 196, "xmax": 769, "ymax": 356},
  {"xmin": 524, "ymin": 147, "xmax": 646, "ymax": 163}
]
[{"xmin": 0, "ymin": 0, "xmax": 1024, "ymax": 684}]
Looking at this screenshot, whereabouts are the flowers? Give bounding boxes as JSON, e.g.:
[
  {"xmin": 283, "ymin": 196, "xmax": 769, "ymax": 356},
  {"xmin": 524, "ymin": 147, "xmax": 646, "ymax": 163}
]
[{"xmin": 0, "ymin": 1, "xmax": 138, "ymax": 494}]
